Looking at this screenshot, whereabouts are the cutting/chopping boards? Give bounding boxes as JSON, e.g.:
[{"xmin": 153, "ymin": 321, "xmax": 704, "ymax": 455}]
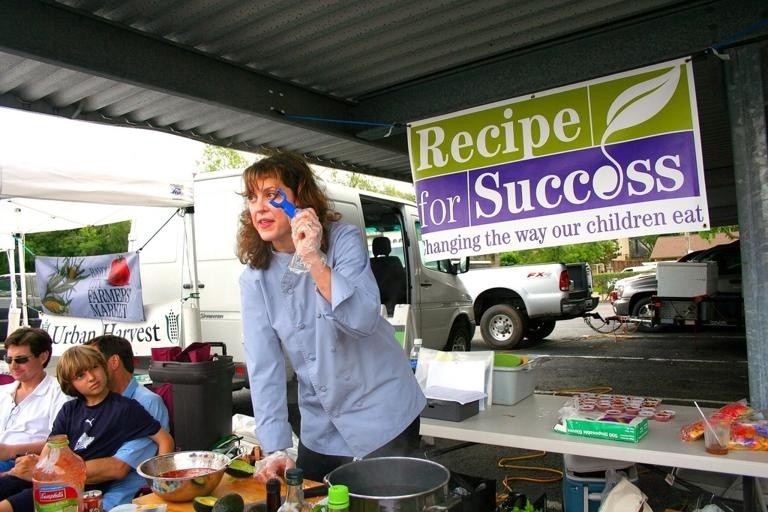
[{"xmin": 131, "ymin": 471, "xmax": 325, "ymax": 512}]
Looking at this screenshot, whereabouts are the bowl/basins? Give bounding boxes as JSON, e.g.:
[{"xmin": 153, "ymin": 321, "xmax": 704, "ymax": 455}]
[
  {"xmin": 494, "ymin": 354, "xmax": 529, "ymax": 368},
  {"xmin": 137, "ymin": 450, "xmax": 231, "ymax": 503},
  {"xmin": 578, "ymin": 392, "xmax": 676, "ymax": 420}
]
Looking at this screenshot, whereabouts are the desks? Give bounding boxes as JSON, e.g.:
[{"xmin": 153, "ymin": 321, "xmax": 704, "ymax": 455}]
[{"xmin": 418, "ymin": 396, "xmax": 767, "ymax": 510}]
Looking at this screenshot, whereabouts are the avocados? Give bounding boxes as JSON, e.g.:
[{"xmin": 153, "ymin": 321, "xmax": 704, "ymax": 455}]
[
  {"xmin": 193, "ymin": 495, "xmax": 217, "ymax": 512},
  {"xmin": 212, "ymin": 493, "xmax": 244, "ymax": 511}
]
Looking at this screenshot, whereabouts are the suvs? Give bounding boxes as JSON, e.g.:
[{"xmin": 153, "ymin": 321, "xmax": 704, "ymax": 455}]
[{"xmin": 0, "ymin": 273, "xmax": 41, "ymax": 343}]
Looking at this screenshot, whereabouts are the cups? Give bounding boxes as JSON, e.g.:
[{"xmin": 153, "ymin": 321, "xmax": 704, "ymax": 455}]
[{"xmin": 704, "ymin": 413, "xmax": 732, "ymax": 455}]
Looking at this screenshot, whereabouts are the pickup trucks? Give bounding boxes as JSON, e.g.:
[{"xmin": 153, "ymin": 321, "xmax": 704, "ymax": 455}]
[{"xmin": 457, "ymin": 260, "xmax": 600, "ymax": 354}]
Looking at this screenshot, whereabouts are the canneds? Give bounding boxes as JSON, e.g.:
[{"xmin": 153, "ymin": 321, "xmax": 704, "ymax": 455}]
[{"xmin": 78, "ymin": 489, "xmax": 103, "ymax": 511}]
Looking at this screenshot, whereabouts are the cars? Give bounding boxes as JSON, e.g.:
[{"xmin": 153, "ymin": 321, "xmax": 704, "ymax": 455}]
[{"xmin": 611, "ymin": 239, "xmax": 743, "ymax": 332}]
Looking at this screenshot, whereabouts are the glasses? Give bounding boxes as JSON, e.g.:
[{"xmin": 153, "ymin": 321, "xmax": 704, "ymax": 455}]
[{"xmin": 4, "ymin": 352, "xmax": 42, "ymax": 364}]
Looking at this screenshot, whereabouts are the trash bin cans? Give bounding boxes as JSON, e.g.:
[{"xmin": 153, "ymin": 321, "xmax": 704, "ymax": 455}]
[{"xmin": 147, "ymin": 355, "xmax": 235, "ymax": 452}]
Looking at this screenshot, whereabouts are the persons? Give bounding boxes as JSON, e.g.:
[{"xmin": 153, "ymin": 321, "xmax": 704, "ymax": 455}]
[
  {"xmin": 236, "ymin": 149, "xmax": 429, "ymax": 482},
  {"xmin": 0, "ymin": 327, "xmax": 79, "ymax": 474}
]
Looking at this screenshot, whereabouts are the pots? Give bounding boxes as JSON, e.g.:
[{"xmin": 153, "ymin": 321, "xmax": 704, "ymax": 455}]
[{"xmin": 322, "ymin": 456, "xmax": 462, "ymax": 511}]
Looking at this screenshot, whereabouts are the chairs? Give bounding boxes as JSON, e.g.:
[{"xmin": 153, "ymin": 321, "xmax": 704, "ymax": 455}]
[{"xmin": 134, "ymin": 383, "xmax": 174, "ymax": 498}]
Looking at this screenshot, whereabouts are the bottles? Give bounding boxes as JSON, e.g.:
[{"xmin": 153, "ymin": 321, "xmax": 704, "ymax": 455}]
[
  {"xmin": 277, "ymin": 468, "xmax": 304, "ymax": 512},
  {"xmin": 409, "ymin": 338, "xmax": 423, "ymax": 373},
  {"xmin": 266, "ymin": 478, "xmax": 282, "ymax": 512},
  {"xmin": 325, "ymin": 484, "xmax": 351, "ymax": 512},
  {"xmin": 32, "ymin": 434, "xmax": 87, "ymax": 512}
]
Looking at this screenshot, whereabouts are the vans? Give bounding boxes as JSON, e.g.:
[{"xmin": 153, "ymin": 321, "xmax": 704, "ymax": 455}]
[{"xmin": 128, "ymin": 166, "xmax": 475, "ymax": 394}]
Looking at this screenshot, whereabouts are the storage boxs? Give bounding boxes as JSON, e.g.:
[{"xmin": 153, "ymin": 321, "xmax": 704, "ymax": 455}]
[
  {"xmin": 657, "ymin": 262, "xmax": 718, "ymax": 297},
  {"xmin": 493, "ymin": 368, "xmax": 536, "ymax": 405}
]
